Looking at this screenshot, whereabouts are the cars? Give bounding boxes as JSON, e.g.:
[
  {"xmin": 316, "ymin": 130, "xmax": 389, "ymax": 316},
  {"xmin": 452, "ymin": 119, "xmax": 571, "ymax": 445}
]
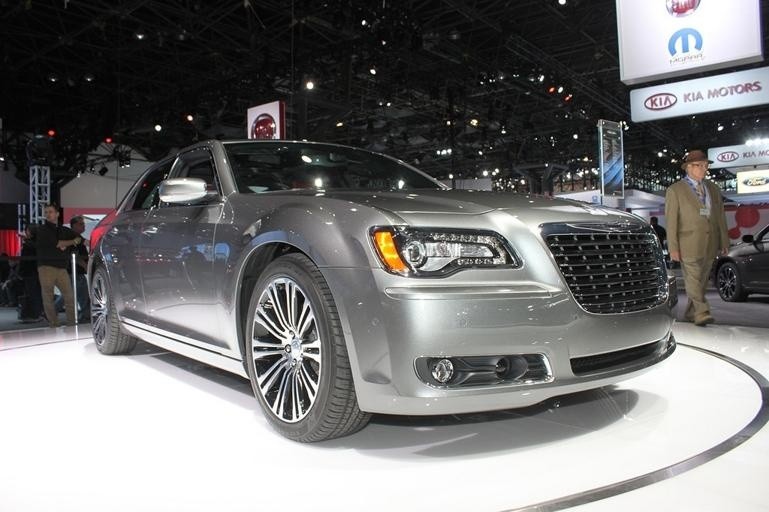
[
  {"xmin": 707, "ymin": 223, "xmax": 768, "ymax": 304},
  {"xmin": 79, "ymin": 137, "xmax": 681, "ymax": 457}
]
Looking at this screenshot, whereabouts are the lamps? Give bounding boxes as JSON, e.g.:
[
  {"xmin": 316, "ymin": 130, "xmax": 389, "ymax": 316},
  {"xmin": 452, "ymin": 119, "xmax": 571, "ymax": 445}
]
[{"xmin": 98, "ymin": 156, "xmax": 131, "ymax": 176}]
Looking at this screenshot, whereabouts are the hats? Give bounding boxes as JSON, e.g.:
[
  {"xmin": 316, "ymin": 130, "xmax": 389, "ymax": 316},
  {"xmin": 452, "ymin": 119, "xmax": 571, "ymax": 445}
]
[{"xmin": 681, "ymin": 151, "xmax": 713, "ymax": 170}]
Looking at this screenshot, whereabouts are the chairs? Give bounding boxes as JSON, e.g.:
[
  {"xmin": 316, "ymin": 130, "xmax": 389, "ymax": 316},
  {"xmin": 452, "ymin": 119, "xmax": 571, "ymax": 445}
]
[{"xmin": 234, "ymin": 160, "xmax": 348, "ymax": 198}]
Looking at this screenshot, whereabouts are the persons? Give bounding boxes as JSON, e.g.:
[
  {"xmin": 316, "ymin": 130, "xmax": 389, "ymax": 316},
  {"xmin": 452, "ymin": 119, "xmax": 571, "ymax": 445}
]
[
  {"xmin": 17, "ymin": 223, "xmax": 48, "ymax": 323},
  {"xmin": 0, "ymin": 252, "xmax": 12, "ymax": 307},
  {"xmin": 51, "ymin": 216, "xmax": 92, "ymax": 323},
  {"xmin": 649, "ymin": 217, "xmax": 667, "ymax": 247},
  {"xmin": 34, "ymin": 203, "xmax": 82, "ymax": 329},
  {"xmin": 1, "ymin": 256, "xmax": 23, "ymax": 307},
  {"xmin": 663, "ymin": 149, "xmax": 730, "ymax": 327}
]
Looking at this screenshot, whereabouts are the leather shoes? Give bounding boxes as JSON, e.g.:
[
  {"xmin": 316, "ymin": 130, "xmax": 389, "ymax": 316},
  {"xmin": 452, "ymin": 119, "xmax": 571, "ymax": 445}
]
[{"xmin": 684, "ymin": 311, "xmax": 713, "ymax": 326}]
